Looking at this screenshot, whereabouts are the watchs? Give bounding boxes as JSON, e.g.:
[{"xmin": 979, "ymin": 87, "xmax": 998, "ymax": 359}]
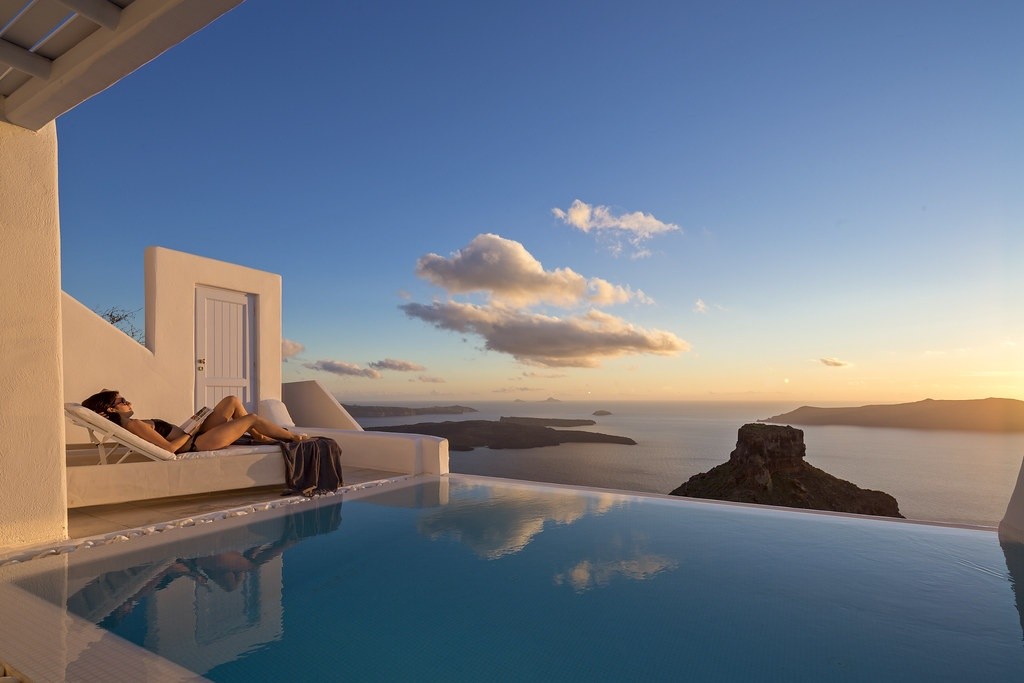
[{"xmin": 186, "ymin": 432, "xmax": 193, "ymax": 439}]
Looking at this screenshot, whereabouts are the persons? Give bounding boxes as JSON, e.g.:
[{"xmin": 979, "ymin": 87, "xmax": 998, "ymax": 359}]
[
  {"xmin": 81, "ymin": 388, "xmax": 308, "ymax": 453},
  {"xmin": 107, "ymin": 538, "xmax": 299, "ymax": 621}
]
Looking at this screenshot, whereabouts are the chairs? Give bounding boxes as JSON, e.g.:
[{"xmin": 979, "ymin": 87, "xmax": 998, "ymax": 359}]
[{"xmin": 64, "ymin": 403, "xmax": 337, "ymax": 466}]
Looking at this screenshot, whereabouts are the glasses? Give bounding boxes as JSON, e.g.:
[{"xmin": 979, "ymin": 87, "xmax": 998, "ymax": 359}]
[{"xmin": 112, "ymin": 398, "xmax": 127, "ymax": 408}]
